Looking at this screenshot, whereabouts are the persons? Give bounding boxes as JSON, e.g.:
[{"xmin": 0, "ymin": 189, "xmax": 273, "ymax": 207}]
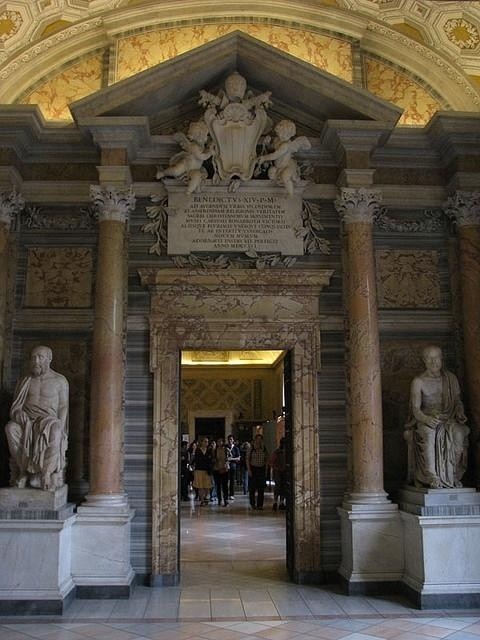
[
  {"xmin": 180, "ymin": 434, "xmax": 285, "ymax": 512},
  {"xmin": 256, "ymin": 120, "xmax": 300, "ymax": 197},
  {"xmin": 4, "ymin": 345, "xmax": 69, "ymax": 490},
  {"xmin": 409, "ymin": 344, "xmax": 472, "ymax": 489},
  {"xmin": 155, "ymin": 122, "xmax": 214, "ymax": 195}
]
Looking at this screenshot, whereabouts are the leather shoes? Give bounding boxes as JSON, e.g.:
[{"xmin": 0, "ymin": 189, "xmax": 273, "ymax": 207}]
[{"xmin": 273, "ymin": 503, "xmax": 285, "ymax": 509}]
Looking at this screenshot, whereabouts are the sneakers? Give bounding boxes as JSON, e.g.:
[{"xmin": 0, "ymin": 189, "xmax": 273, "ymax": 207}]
[{"xmin": 218, "ymin": 499, "xmax": 230, "ymax": 506}]
